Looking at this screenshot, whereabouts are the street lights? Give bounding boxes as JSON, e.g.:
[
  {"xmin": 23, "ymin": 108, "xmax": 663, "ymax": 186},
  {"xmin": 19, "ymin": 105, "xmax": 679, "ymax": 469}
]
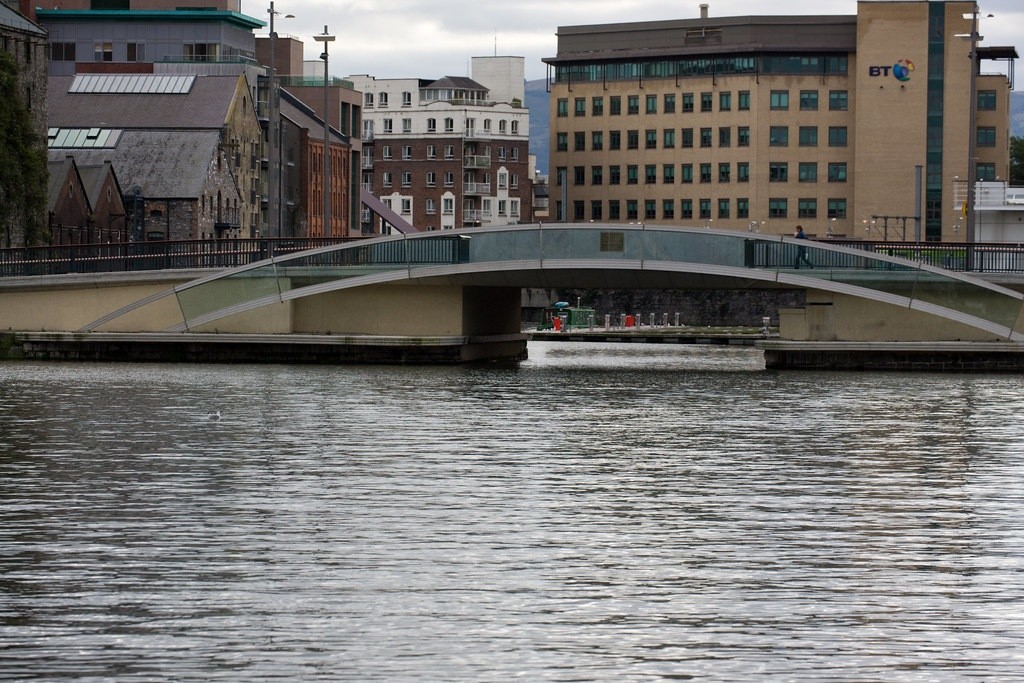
[
  {"xmin": 267, "ymin": 1, "xmax": 296, "ymax": 269},
  {"xmin": 828, "ymin": 217, "xmax": 836, "ymax": 239},
  {"xmin": 311, "ymin": 24, "xmax": 337, "ymax": 267},
  {"xmin": 862, "ymin": 219, "xmax": 876, "ymax": 239}
]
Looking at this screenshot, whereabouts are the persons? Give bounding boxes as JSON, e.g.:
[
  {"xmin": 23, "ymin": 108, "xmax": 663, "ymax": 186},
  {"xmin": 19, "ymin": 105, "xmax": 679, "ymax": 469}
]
[{"xmin": 794, "ymin": 225, "xmax": 813, "ymax": 269}]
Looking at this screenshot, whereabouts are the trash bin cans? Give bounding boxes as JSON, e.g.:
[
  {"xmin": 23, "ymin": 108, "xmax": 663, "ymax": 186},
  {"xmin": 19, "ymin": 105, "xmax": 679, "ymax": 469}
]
[
  {"xmin": 260, "ymin": 235, "xmax": 273, "ymax": 260},
  {"xmin": 146, "ymin": 230, "xmax": 165, "ymax": 269}
]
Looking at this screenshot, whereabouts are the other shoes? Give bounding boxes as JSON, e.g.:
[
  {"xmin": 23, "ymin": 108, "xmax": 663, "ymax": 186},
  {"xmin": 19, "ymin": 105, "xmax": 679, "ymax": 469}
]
[
  {"xmin": 795, "ymin": 267, "xmax": 799, "ymax": 269},
  {"xmin": 810, "ymin": 263, "xmax": 813, "ymax": 269}
]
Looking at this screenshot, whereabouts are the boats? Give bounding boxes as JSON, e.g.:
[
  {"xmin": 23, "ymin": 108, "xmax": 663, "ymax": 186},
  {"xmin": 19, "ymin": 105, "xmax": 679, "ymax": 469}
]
[{"xmin": 536, "ymin": 296, "xmax": 595, "ymax": 332}]
